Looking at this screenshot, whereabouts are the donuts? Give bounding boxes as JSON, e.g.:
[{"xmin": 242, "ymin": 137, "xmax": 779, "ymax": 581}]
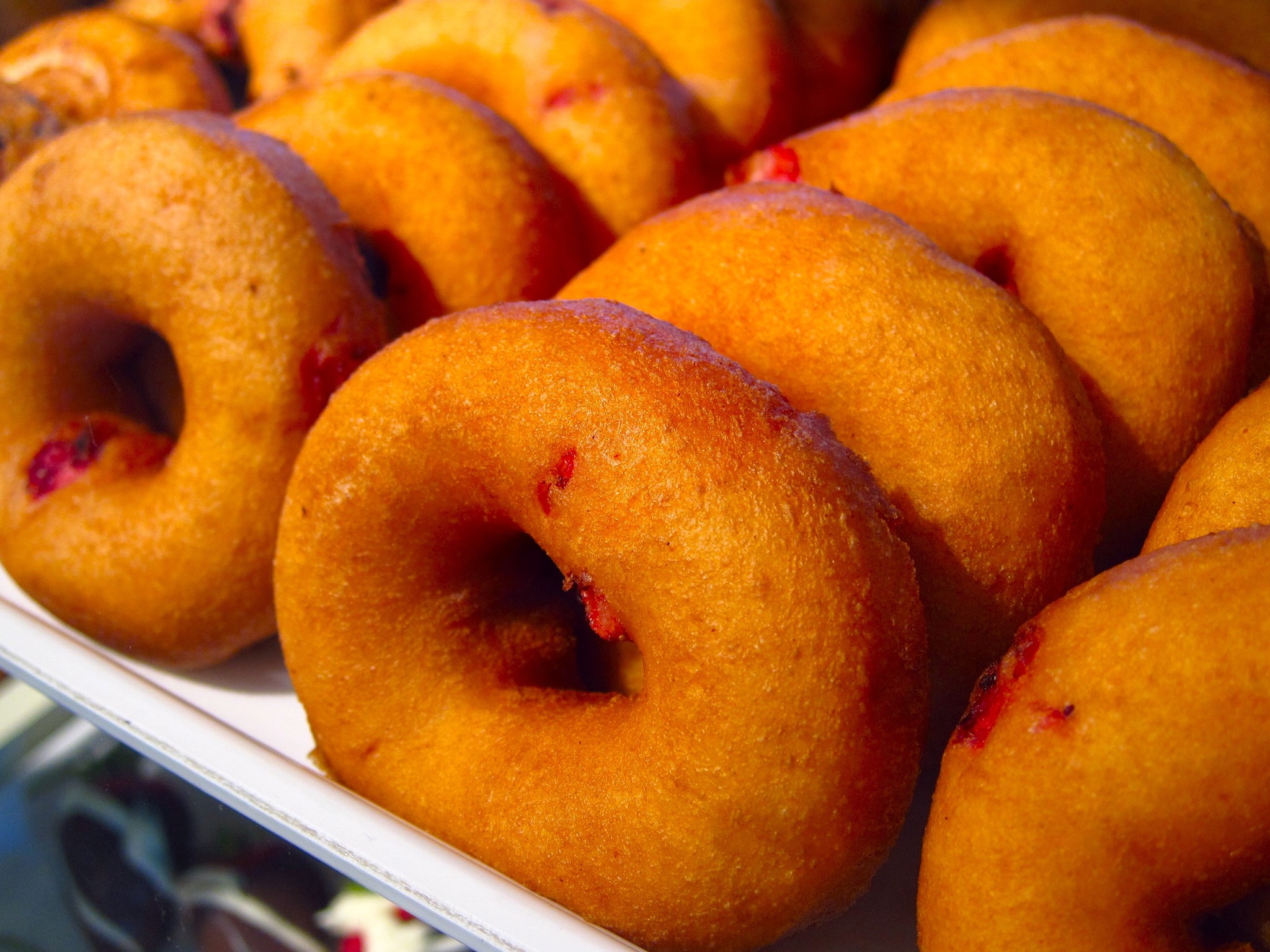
[{"xmin": 0, "ymin": 0, "xmax": 1270, "ymax": 951}]
